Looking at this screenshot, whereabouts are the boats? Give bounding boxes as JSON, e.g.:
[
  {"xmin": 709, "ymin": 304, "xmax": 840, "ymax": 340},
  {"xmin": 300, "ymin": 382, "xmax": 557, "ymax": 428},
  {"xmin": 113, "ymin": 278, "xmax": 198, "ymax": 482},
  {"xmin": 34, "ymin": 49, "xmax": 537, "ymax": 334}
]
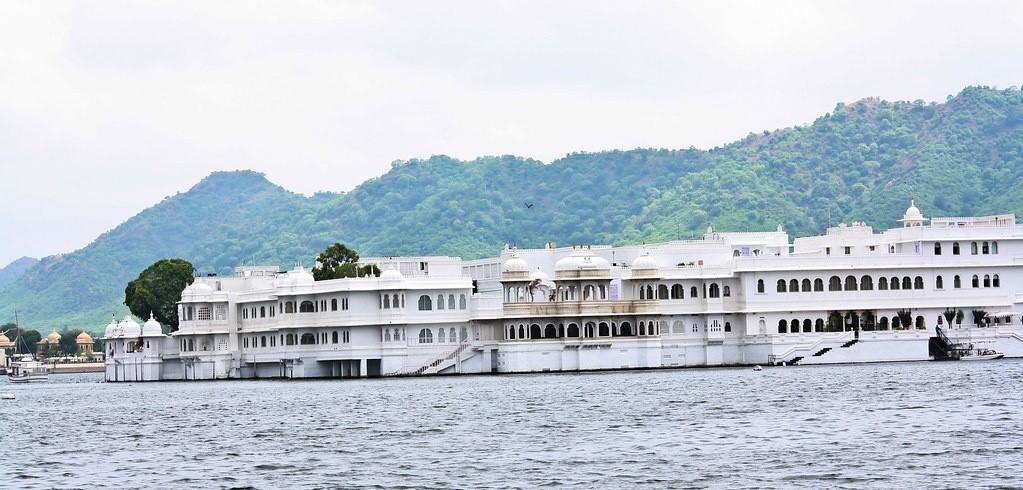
[
  {"xmin": 959, "ymin": 350, "xmax": 1005, "ymax": 361},
  {"xmin": 1, "ymin": 392, "xmax": 15, "ymax": 399}
]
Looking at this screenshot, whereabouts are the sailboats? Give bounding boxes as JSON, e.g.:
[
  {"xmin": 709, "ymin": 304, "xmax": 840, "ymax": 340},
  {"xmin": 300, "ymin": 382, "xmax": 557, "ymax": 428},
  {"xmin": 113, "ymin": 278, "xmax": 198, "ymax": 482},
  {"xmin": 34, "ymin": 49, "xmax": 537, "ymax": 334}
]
[{"xmin": 7, "ymin": 311, "xmax": 48, "ymax": 383}]
